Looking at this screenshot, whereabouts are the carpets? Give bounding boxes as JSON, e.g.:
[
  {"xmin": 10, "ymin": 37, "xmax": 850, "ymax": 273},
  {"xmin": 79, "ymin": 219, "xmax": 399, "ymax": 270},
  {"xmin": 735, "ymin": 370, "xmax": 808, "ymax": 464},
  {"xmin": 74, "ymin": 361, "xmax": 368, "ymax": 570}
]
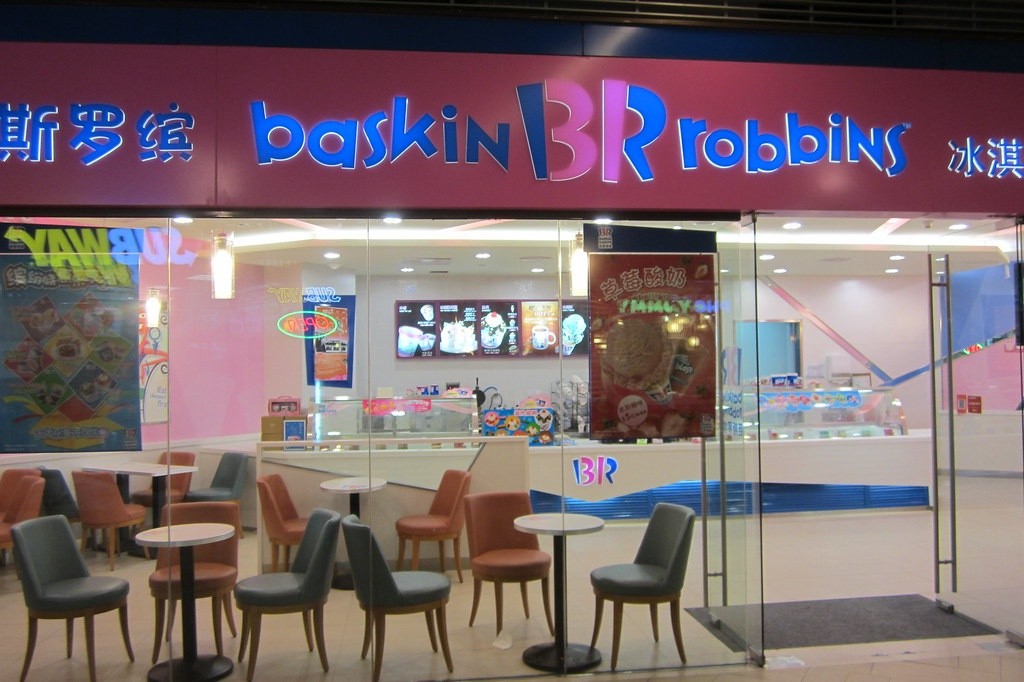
[{"xmin": 683, "ymin": 593, "xmax": 1005, "ymax": 653}]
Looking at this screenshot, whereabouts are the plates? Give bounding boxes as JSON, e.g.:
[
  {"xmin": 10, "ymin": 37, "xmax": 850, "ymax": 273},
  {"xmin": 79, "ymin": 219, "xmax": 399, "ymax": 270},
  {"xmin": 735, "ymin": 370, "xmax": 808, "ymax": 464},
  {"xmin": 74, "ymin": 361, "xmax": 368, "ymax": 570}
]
[{"xmin": 440, "ymin": 341, "xmax": 477, "ymax": 353}]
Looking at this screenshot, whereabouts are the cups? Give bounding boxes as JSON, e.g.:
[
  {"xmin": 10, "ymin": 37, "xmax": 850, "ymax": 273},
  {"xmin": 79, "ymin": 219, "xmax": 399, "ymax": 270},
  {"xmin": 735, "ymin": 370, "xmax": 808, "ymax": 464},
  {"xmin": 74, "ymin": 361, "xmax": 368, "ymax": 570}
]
[
  {"xmin": 669, "ymin": 339, "xmax": 709, "ymax": 399},
  {"xmin": 398, "ymin": 325, "xmax": 423, "ymax": 357},
  {"xmin": 563, "ymin": 342, "xmax": 577, "ymax": 355},
  {"xmin": 771, "ymin": 375, "xmax": 787, "ymax": 386},
  {"xmin": 644, "ymin": 375, "xmax": 673, "ymax": 405},
  {"xmin": 425, "ymin": 333, "xmax": 437, "ymax": 350},
  {"xmin": 374, "ymin": 386, "xmax": 395, "ymax": 400},
  {"xmin": 430, "ymin": 385, "xmax": 439, "ymax": 396},
  {"xmin": 532, "ymin": 325, "xmax": 556, "ymax": 350},
  {"xmin": 787, "ymin": 373, "xmax": 798, "ymax": 386},
  {"xmin": 557, "ymin": 374, "xmax": 590, "ymax": 433},
  {"xmin": 420, "ymin": 305, "xmax": 434, "ymax": 322},
  {"xmin": 416, "ymin": 386, "xmax": 429, "ymax": 396},
  {"xmin": 418, "ymin": 335, "xmax": 429, "ymax": 351}
]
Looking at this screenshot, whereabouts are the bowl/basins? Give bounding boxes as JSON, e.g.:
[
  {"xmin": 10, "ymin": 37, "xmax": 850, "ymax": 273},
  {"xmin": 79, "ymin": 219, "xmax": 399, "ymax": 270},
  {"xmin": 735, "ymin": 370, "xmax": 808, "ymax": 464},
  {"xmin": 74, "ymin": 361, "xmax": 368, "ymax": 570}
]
[{"xmin": 481, "ymin": 316, "xmax": 507, "ymax": 349}]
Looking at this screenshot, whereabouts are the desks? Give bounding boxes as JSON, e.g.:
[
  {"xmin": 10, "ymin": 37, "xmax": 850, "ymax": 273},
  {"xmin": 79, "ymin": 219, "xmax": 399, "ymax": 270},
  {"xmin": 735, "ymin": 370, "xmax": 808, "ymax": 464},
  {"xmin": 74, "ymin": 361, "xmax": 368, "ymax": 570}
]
[
  {"xmin": 514, "ymin": 512, "xmax": 605, "ymax": 675},
  {"xmin": 319, "ymin": 477, "xmax": 387, "ymax": 591},
  {"xmin": 83, "ymin": 462, "xmax": 199, "ymax": 559},
  {"xmin": 135, "ymin": 523, "xmax": 235, "ymax": 682}
]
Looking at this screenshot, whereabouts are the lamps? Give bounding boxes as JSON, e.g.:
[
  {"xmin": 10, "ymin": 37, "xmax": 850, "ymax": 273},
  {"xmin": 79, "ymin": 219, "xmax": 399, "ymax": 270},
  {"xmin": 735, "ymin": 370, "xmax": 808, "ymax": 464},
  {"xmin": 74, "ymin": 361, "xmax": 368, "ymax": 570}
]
[
  {"xmin": 567, "ymin": 231, "xmax": 590, "ymax": 297},
  {"xmin": 210, "ymin": 230, "xmax": 235, "ymax": 300},
  {"xmin": 145, "ymin": 289, "xmax": 162, "ymax": 328}
]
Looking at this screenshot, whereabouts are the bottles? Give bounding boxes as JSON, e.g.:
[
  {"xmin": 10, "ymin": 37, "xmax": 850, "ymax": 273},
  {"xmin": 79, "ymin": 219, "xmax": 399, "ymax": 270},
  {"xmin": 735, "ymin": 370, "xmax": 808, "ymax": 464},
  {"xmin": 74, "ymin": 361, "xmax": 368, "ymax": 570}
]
[{"xmin": 899, "ymin": 407, "xmax": 910, "ymax": 436}]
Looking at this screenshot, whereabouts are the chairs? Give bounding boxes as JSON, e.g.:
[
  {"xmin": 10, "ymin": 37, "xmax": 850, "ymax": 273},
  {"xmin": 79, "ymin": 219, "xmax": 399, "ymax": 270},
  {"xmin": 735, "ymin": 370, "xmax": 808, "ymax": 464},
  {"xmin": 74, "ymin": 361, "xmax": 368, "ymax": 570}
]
[
  {"xmin": 234, "ymin": 508, "xmax": 340, "ymax": 682},
  {"xmin": 342, "ymin": 514, "xmax": 454, "ymax": 682},
  {"xmin": 0, "ymin": 466, "xmax": 81, "ymax": 579},
  {"xmin": 590, "ymin": 502, "xmax": 695, "ymax": 673},
  {"xmin": 395, "ymin": 469, "xmax": 471, "ymax": 583},
  {"xmin": 11, "ymin": 515, "xmax": 135, "ymax": 682},
  {"xmin": 72, "ymin": 471, "xmax": 150, "ymax": 572},
  {"xmin": 464, "ymin": 492, "xmax": 555, "ymax": 642},
  {"xmin": 185, "ymin": 452, "xmax": 248, "ymax": 539},
  {"xmin": 131, "ymin": 452, "xmax": 195, "ymax": 540},
  {"xmin": 256, "ymin": 474, "xmax": 309, "ymax": 573},
  {"xmin": 149, "ymin": 502, "xmax": 240, "ymax": 665}
]
